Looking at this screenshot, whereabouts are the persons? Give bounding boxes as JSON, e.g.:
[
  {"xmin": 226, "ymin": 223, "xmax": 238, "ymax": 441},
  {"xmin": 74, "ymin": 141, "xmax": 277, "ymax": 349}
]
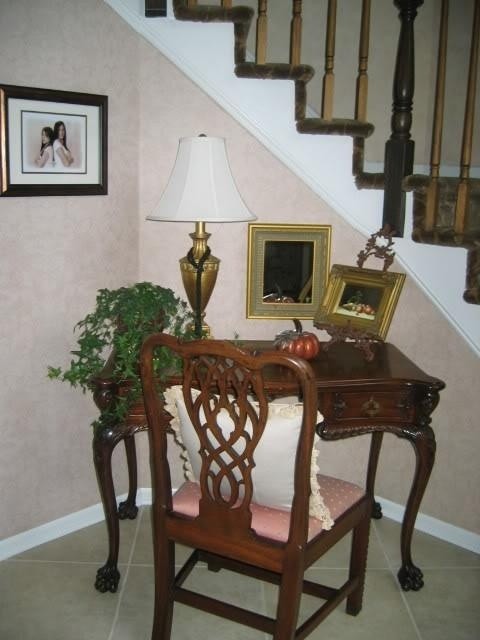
[
  {"xmin": 35, "ymin": 127, "xmax": 55, "ymax": 169},
  {"xmin": 53, "ymin": 121, "xmax": 74, "ymax": 169}
]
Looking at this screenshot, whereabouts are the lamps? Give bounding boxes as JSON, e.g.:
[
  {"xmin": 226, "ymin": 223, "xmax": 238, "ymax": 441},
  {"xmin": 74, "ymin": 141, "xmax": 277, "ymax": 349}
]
[{"xmin": 145, "ymin": 137, "xmax": 258, "ymax": 337}]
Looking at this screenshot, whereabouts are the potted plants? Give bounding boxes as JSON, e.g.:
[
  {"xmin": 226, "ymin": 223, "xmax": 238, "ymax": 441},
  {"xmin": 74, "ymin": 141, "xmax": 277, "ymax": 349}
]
[{"xmin": 46, "ymin": 282, "xmax": 245, "ymax": 427}]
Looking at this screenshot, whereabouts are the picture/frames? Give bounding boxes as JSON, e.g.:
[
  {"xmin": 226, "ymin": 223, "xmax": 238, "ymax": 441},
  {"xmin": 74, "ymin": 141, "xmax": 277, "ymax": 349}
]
[
  {"xmin": 0, "ymin": 84, "xmax": 108, "ymax": 197},
  {"xmin": 313, "ymin": 264, "xmax": 406, "ymax": 340},
  {"xmin": 246, "ymin": 223, "xmax": 333, "ymax": 320}
]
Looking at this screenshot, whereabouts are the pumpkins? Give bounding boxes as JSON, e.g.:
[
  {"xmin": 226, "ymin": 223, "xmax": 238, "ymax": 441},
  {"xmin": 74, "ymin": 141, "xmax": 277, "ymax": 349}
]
[{"xmin": 274, "ymin": 318, "xmax": 320, "ymax": 360}]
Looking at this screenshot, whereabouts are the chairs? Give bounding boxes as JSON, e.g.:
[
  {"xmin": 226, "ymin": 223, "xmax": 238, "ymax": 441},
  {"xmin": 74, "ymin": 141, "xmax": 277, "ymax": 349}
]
[{"xmin": 141, "ymin": 334, "xmax": 372, "ymax": 640}]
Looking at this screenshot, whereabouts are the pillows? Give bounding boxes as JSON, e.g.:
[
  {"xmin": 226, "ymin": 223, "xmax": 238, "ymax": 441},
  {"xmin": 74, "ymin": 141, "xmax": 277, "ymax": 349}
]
[
  {"xmin": 163, "ymin": 385, "xmax": 334, "ymax": 531},
  {"xmin": 92, "ymin": 339, "xmax": 446, "ymax": 593}
]
